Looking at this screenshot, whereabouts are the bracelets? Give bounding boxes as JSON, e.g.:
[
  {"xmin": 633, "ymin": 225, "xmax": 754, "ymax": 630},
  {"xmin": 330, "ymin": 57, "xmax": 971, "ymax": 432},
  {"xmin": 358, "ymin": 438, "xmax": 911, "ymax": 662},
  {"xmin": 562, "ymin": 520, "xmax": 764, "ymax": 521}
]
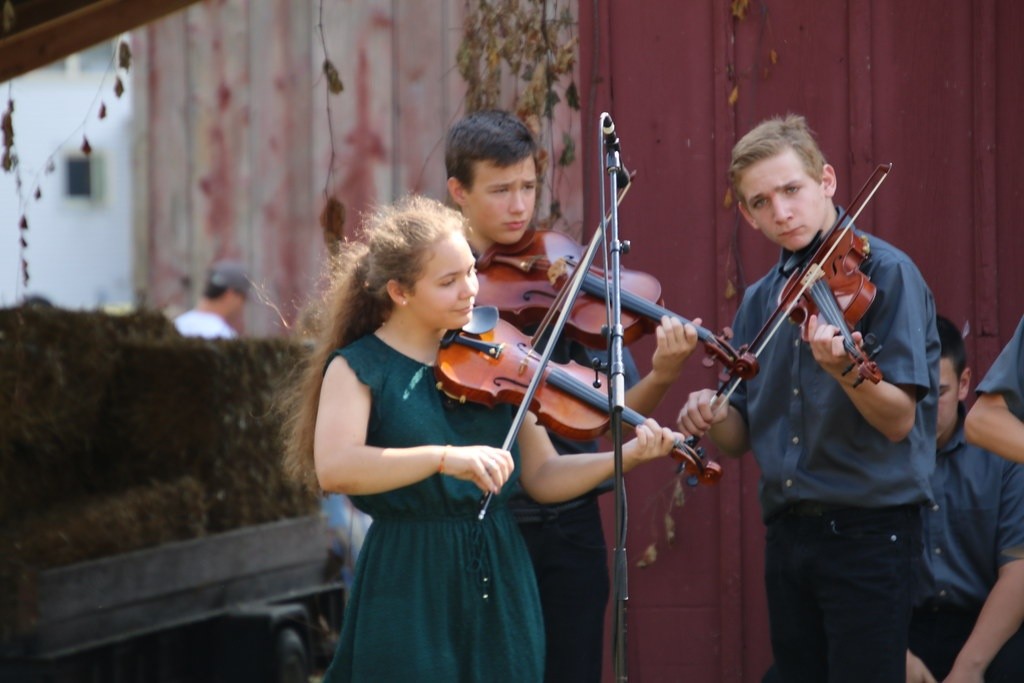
[{"xmin": 437, "ymin": 444, "xmax": 452, "ymax": 474}]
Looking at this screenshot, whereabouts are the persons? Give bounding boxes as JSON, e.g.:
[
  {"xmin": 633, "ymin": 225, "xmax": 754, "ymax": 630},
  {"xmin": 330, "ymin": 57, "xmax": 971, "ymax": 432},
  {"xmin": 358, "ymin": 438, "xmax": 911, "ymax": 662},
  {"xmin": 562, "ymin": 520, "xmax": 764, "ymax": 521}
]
[
  {"xmin": 281, "ymin": 198, "xmax": 685, "ymax": 683},
  {"xmin": 444, "ymin": 106, "xmax": 703, "ymax": 683},
  {"xmin": 905, "ymin": 312, "xmax": 1024, "ymax": 683},
  {"xmin": 677, "ymin": 116, "xmax": 940, "ymax": 683},
  {"xmin": 176, "ymin": 261, "xmax": 256, "ymax": 338}
]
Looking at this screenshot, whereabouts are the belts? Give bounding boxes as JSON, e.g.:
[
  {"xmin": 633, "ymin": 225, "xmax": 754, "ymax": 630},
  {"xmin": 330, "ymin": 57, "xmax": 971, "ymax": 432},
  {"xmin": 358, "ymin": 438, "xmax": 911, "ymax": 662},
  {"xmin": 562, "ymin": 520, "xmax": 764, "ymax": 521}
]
[
  {"xmin": 789, "ymin": 501, "xmax": 839, "ymax": 516},
  {"xmin": 510, "ymin": 493, "xmax": 596, "ymax": 524}
]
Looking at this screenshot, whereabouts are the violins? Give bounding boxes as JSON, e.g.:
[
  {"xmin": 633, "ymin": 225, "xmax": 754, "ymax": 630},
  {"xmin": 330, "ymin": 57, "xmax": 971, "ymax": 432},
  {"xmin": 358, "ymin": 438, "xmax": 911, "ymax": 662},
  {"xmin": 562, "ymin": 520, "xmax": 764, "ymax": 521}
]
[
  {"xmin": 433, "ymin": 313, "xmax": 724, "ymax": 489},
  {"xmin": 472, "ymin": 226, "xmax": 762, "ymax": 381},
  {"xmin": 775, "ymin": 224, "xmax": 884, "ymax": 391}
]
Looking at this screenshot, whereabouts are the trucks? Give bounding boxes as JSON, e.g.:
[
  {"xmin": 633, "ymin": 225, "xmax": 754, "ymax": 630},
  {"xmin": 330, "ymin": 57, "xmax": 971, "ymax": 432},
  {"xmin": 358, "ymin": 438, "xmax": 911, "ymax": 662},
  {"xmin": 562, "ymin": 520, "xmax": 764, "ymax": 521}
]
[{"xmin": 1, "ymin": 490, "xmax": 350, "ymax": 683}]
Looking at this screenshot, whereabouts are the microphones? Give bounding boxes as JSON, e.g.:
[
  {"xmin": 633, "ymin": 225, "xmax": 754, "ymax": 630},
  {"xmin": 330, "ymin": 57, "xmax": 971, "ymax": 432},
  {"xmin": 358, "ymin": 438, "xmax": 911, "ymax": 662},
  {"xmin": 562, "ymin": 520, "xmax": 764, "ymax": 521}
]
[{"xmin": 600, "ymin": 111, "xmax": 630, "ymax": 188}]
